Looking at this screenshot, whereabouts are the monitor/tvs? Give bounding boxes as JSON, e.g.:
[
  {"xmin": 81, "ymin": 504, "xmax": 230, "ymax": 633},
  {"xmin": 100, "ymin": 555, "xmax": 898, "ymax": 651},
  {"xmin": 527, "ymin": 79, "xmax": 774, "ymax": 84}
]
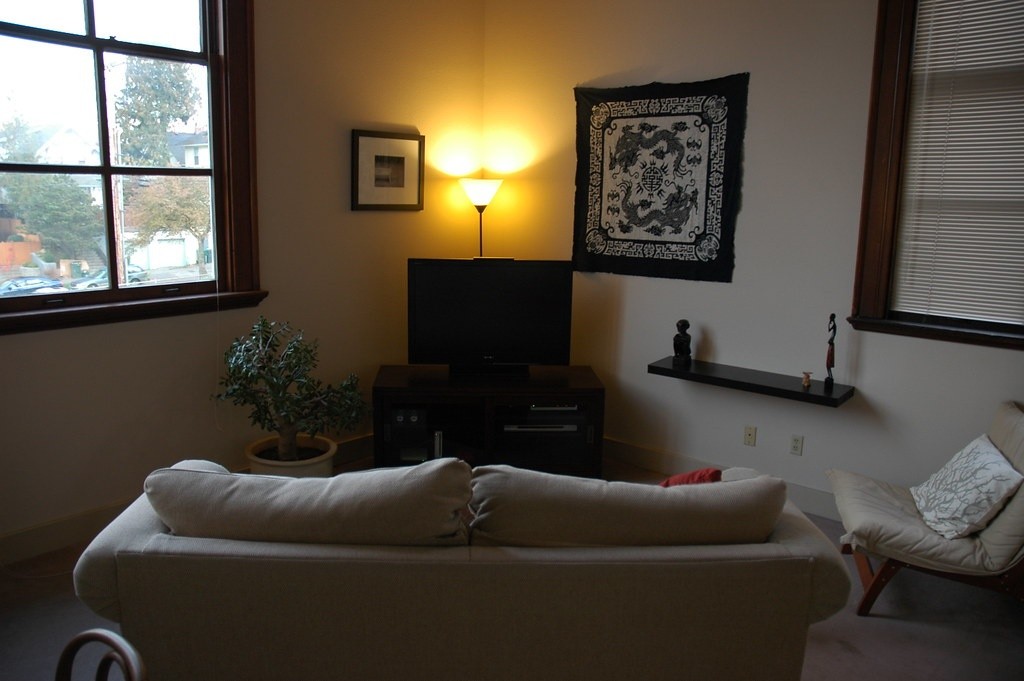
[{"xmin": 407, "ymin": 259, "xmax": 573, "ymax": 381}]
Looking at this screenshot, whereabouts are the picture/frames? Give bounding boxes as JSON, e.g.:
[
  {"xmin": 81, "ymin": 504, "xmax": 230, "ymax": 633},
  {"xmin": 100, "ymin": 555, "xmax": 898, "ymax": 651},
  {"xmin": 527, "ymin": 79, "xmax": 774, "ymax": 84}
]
[
  {"xmin": 571, "ymin": 72, "xmax": 750, "ymax": 284},
  {"xmin": 350, "ymin": 128, "xmax": 425, "ymax": 212}
]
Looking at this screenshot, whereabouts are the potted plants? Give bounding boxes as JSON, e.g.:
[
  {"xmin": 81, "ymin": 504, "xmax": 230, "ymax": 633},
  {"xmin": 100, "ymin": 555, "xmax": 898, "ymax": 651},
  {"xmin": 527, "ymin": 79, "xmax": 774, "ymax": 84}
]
[{"xmin": 211, "ymin": 313, "xmax": 362, "ymax": 478}]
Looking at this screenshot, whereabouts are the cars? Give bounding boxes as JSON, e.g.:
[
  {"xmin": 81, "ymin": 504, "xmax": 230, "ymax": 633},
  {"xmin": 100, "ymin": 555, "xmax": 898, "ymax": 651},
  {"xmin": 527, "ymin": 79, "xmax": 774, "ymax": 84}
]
[
  {"xmin": 32, "ymin": 286, "xmax": 70, "ymax": 295},
  {"xmin": 0, "ymin": 276, "xmax": 64, "ymax": 296},
  {"xmin": 69, "ymin": 265, "xmax": 150, "ymax": 291}
]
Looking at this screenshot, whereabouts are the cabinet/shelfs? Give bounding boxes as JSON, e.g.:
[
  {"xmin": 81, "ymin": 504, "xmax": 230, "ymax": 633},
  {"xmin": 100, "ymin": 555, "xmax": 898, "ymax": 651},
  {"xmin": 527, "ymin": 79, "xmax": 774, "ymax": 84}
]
[{"xmin": 371, "ymin": 365, "xmax": 606, "ymax": 479}]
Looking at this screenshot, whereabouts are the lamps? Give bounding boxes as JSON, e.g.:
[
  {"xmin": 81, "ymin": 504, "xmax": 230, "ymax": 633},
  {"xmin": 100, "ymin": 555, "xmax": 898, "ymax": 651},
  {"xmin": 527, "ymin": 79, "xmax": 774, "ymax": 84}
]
[{"xmin": 460, "ymin": 178, "xmax": 504, "ymax": 257}]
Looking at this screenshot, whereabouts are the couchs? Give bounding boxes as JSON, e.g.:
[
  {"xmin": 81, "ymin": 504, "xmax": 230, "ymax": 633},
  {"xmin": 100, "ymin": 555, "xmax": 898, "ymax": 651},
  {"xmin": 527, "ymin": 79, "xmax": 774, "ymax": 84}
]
[{"xmin": 73, "ymin": 460, "xmax": 850, "ymax": 681}]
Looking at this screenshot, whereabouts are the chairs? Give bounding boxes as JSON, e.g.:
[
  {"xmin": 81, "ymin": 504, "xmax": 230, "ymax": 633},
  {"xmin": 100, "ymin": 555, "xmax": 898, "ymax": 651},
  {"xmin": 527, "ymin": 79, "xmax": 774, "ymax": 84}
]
[{"xmin": 820, "ymin": 400, "xmax": 1024, "ymax": 616}]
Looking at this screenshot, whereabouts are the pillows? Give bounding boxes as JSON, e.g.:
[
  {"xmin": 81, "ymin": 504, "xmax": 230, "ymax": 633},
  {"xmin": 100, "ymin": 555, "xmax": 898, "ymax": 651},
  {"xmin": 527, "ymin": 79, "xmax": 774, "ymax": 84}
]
[
  {"xmin": 143, "ymin": 456, "xmax": 476, "ymax": 545},
  {"xmin": 465, "ymin": 464, "xmax": 787, "ymax": 544},
  {"xmin": 909, "ymin": 434, "xmax": 1024, "ymax": 542}
]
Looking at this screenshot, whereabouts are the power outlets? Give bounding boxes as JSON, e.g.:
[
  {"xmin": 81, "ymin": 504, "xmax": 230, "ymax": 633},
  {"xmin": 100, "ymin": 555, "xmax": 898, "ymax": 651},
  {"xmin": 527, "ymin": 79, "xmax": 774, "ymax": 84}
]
[
  {"xmin": 789, "ymin": 434, "xmax": 804, "ymax": 456},
  {"xmin": 743, "ymin": 426, "xmax": 755, "ymax": 447}
]
